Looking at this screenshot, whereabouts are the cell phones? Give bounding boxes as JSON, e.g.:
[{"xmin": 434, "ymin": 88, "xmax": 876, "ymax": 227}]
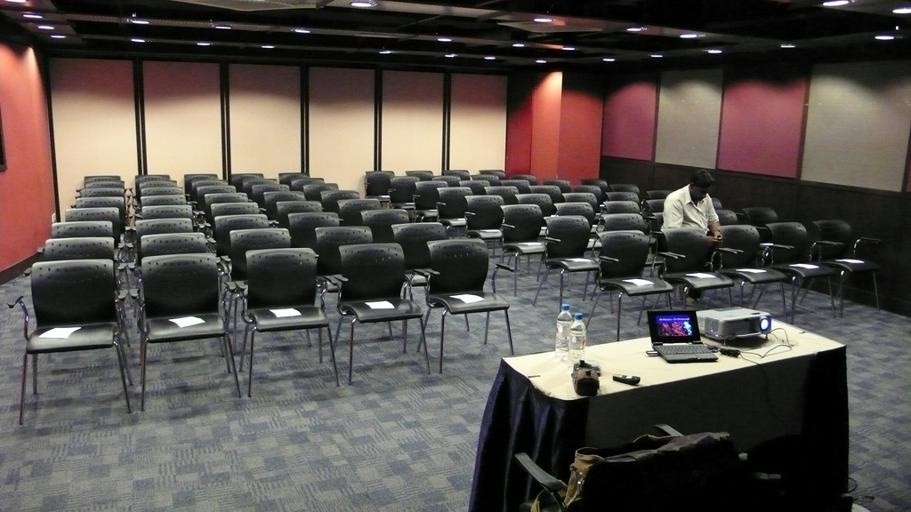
[{"xmin": 613, "ymin": 374, "xmax": 640, "ymax": 385}]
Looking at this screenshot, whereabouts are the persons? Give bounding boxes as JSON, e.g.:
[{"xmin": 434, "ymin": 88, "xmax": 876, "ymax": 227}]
[{"xmin": 661, "ymin": 170, "xmax": 723, "ymax": 311}]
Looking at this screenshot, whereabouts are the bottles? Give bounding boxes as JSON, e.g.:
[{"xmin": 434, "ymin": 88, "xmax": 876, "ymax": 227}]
[
  {"xmin": 555, "ymin": 304, "xmax": 573, "ymax": 362},
  {"xmin": 569, "ymin": 312, "xmax": 586, "ymax": 372}
]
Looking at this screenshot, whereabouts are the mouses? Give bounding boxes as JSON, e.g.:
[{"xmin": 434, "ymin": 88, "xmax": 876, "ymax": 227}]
[{"xmin": 720, "ymin": 345, "xmax": 741, "ymax": 357}]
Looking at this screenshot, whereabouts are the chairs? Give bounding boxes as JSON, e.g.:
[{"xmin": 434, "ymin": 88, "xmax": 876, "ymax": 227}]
[
  {"xmin": 77, "ymin": 188, "xmax": 132, "ymax": 196},
  {"xmin": 222, "ymin": 228, "xmax": 291, "ymax": 283},
  {"xmin": 261, "ymin": 191, "xmax": 307, "ymax": 216},
  {"xmin": 199, "ymin": 193, "xmax": 249, "ymax": 217},
  {"xmin": 432, "ymin": 176, "xmax": 462, "ymax": 187},
  {"xmin": 498, "ymin": 203, "xmax": 546, "ymax": 298},
  {"xmin": 407, "ymin": 170, "xmax": 434, "ymax": 181},
  {"xmin": 652, "ymin": 229, "xmax": 735, "ymax": 311},
  {"xmin": 646, "ymin": 199, "xmax": 670, "ymax": 210},
  {"xmin": 647, "ymin": 189, "xmax": 670, "ymax": 198},
  {"xmin": 591, "ymin": 213, "xmax": 648, "ymax": 257},
  {"xmin": 464, "ymin": 194, "xmax": 505, "ymax": 259},
  {"xmin": 242, "ymin": 248, "xmax": 333, "ymax": 345},
  {"xmin": 138, "ymin": 181, "xmax": 178, "ymax": 188},
  {"xmin": 443, "ymin": 170, "xmax": 470, "ymax": 180},
  {"xmin": 553, "ymin": 203, "xmax": 596, "ymax": 222},
  {"xmin": 279, "ymin": 173, "xmax": 310, "ymax": 185},
  {"xmin": 314, "ymin": 225, "xmax": 374, "ymax": 285},
  {"xmin": 250, "ymin": 184, "xmax": 290, "ymax": 200},
  {"xmin": 607, "ymin": 183, "xmax": 640, "ymax": 193},
  {"xmin": 543, "ymin": 179, "xmax": 571, "ymax": 193},
  {"xmin": 132, "ymin": 175, "xmax": 170, "ymax": 200},
  {"xmin": 136, "ymin": 219, "xmax": 207, "ymax": 233},
  {"xmin": 287, "ymin": 212, "xmax": 340, "ymax": 248},
  {"xmin": 391, "ymin": 222, "xmax": 449, "ymax": 300},
  {"xmin": 710, "ymin": 198, "xmax": 723, "ymax": 208},
  {"xmin": 208, "ymin": 214, "xmax": 270, "ymax": 257},
  {"xmin": 320, "ymin": 190, "xmax": 360, "ymax": 211},
  {"xmin": 85, "ymin": 176, "xmax": 122, "ymax": 181},
  {"xmin": 586, "ymin": 230, "xmax": 674, "ymax": 342},
  {"xmin": 138, "ymin": 187, "xmax": 187, "ymax": 195},
  {"xmin": 800, "ymin": 218, "xmax": 881, "ymax": 319},
  {"xmin": 602, "ymin": 200, "xmax": 640, "ymax": 213},
  {"xmin": 192, "ymin": 186, "xmax": 236, "ymax": 210},
  {"xmin": 363, "ymin": 171, "xmax": 395, "ymax": 201},
  {"xmin": 185, "ymin": 174, "xmax": 219, "ymax": 200},
  {"xmin": 134, "ymin": 204, "xmax": 200, "ymax": 224},
  {"xmin": 581, "ymin": 179, "xmax": 607, "ymax": 188},
  {"xmin": 130, "ymin": 252, "xmax": 237, "ymax": 340},
  {"xmin": 412, "ymin": 181, "xmax": 449, "ymax": 223},
  {"xmin": 361, "ymin": 209, "xmax": 410, "ymax": 243},
  {"xmin": 230, "ymin": 173, "xmax": 263, "ymax": 188},
  {"xmin": 468, "ymin": 176, "xmax": 499, "ymax": 187},
  {"xmin": 337, "ymin": 199, "xmax": 382, "ymax": 225},
  {"xmin": 514, "ymin": 423, "xmax": 749, "ymax": 512},
  {"xmin": 304, "ymin": 184, "xmax": 340, "ymax": 201},
  {"xmin": 485, "ymin": 186, "xmax": 520, "ymax": 205},
  {"xmin": 388, "ymin": 177, "xmax": 423, "ymax": 222},
  {"xmin": 7, "ymin": 259, "xmax": 128, "ymax": 340},
  {"xmin": 459, "ymin": 180, "xmax": 491, "ymax": 196},
  {"xmin": 716, "ymin": 224, "xmax": 789, "ymax": 319},
  {"xmin": 418, "ymin": 238, "xmax": 513, "ymax": 347},
  {"xmin": 139, "ymin": 195, "xmax": 194, "ymax": 210},
  {"xmin": 273, "ymin": 201, "xmax": 324, "ymax": 226},
  {"xmin": 500, "ymin": 180, "xmax": 530, "ymax": 194},
  {"xmin": 753, "ymin": 221, "xmax": 838, "ymax": 321},
  {"xmin": 140, "ymin": 230, "xmax": 220, "ymax": 265},
  {"xmin": 204, "ymin": 202, "xmax": 259, "ymax": 227},
  {"xmin": 334, "ymin": 242, "xmax": 426, "ymax": 346},
  {"xmin": 561, "ymin": 191, "xmax": 597, "ymax": 210},
  {"xmin": 71, "ymin": 197, "xmax": 131, "ymax": 208},
  {"xmin": 187, "ymin": 179, "xmax": 228, "ymax": 202},
  {"xmin": 51, "ymin": 221, "xmax": 121, "ymax": 249},
  {"xmin": 513, "ymin": 175, "xmax": 536, "ymax": 184},
  {"xmin": 572, "ymin": 184, "xmax": 601, "ymax": 196},
  {"xmin": 715, "ymin": 209, "xmax": 738, "ymax": 224},
  {"xmin": 65, "ymin": 208, "xmax": 129, "ymax": 221},
  {"xmin": 479, "ymin": 169, "xmax": 506, "ymax": 181},
  {"xmin": 515, "ymin": 193, "xmax": 553, "ymax": 215},
  {"xmin": 290, "ymin": 178, "xmax": 325, "ymax": 191},
  {"xmin": 243, "ymin": 179, "xmax": 277, "ymax": 193},
  {"xmin": 435, "ymin": 187, "xmax": 473, "ymax": 232},
  {"xmin": 84, "ymin": 181, "xmax": 128, "ymax": 187},
  {"xmin": 45, "ymin": 238, "xmax": 123, "ymax": 261},
  {"xmin": 606, "ymin": 191, "xmax": 642, "ymax": 203},
  {"xmin": 532, "ymin": 216, "xmax": 601, "ymax": 308},
  {"xmin": 743, "ymin": 206, "xmax": 777, "ymax": 224}
]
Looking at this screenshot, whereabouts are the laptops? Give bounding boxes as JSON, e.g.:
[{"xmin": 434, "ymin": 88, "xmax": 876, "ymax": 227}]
[{"xmin": 647, "ymin": 309, "xmax": 719, "ymax": 364}]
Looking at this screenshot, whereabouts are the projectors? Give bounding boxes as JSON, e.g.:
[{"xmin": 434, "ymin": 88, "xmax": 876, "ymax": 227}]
[{"xmin": 696, "ymin": 306, "xmax": 772, "ymax": 346}]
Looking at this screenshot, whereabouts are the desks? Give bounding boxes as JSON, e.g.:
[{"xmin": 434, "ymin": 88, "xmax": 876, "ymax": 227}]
[{"xmin": 495, "ymin": 318, "xmax": 849, "ymax": 511}]
